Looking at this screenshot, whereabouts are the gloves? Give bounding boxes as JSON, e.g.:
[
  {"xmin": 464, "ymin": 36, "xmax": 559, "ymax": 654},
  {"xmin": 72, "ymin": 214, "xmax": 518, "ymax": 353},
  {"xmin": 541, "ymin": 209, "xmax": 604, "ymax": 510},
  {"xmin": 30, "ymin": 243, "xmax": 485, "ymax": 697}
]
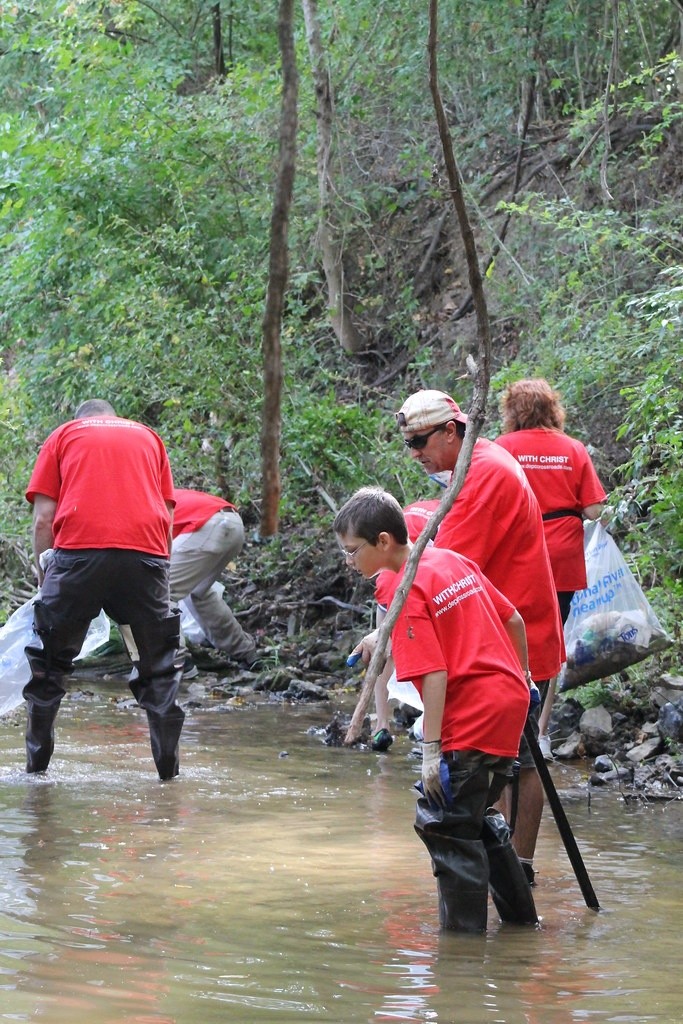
[
  {"xmin": 420, "ymin": 739, "xmax": 452, "ymax": 811},
  {"xmin": 346, "ymin": 627, "xmax": 394, "ymax": 676}
]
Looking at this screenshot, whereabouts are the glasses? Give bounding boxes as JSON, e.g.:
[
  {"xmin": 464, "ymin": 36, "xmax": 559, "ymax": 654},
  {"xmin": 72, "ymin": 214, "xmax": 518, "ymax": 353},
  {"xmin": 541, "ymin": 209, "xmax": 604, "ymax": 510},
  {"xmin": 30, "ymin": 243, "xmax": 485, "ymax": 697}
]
[
  {"xmin": 404, "ymin": 427, "xmax": 442, "ymax": 449},
  {"xmin": 341, "ymin": 533, "xmax": 379, "ymax": 560}
]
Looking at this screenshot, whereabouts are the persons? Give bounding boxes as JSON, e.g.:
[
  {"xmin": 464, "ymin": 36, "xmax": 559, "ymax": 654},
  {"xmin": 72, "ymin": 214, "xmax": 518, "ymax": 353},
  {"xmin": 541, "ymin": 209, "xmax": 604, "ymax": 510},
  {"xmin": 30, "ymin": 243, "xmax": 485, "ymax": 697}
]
[
  {"xmin": 170, "ymin": 489, "xmax": 263, "ymax": 678},
  {"xmin": 346, "ymin": 389, "xmax": 567, "ymax": 883},
  {"xmin": 368, "ymin": 380, "xmax": 606, "ymax": 760},
  {"xmin": 22, "ymin": 399, "xmax": 186, "ymax": 781},
  {"xmin": 333, "ymin": 488, "xmax": 538, "ymax": 933}
]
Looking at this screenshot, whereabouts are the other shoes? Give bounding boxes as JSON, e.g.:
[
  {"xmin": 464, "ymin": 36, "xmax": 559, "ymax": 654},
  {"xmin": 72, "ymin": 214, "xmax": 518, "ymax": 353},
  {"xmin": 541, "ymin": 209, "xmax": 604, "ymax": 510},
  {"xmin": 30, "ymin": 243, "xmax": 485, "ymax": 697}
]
[
  {"xmin": 537, "ymin": 733, "xmax": 553, "ymax": 760},
  {"xmin": 239, "ymin": 650, "xmax": 262, "ymax": 670},
  {"xmin": 180, "ymin": 655, "xmax": 199, "ymax": 680},
  {"xmin": 370, "ymin": 727, "xmax": 391, "ymax": 751}
]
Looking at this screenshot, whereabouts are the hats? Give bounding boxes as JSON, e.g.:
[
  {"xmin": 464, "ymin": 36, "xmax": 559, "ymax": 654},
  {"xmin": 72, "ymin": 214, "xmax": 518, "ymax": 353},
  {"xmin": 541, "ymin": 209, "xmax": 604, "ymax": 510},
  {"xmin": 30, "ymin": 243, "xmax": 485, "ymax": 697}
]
[{"xmin": 393, "ymin": 389, "xmax": 467, "ymax": 432}]
